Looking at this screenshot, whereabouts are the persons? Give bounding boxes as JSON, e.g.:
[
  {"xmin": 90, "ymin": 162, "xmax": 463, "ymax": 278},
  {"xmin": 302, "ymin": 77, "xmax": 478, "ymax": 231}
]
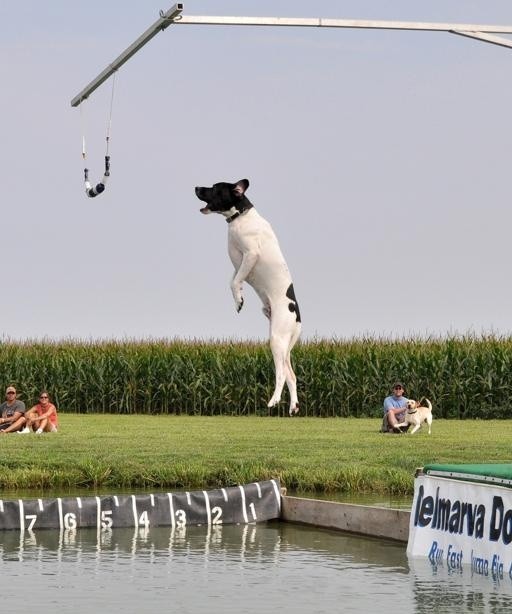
[
  {"xmin": 0, "ymin": 390, "xmax": 58, "ymax": 434},
  {"xmin": 0, "ymin": 386, "xmax": 26, "ymax": 432},
  {"xmin": 380, "ymin": 382, "xmax": 411, "ymax": 434}
]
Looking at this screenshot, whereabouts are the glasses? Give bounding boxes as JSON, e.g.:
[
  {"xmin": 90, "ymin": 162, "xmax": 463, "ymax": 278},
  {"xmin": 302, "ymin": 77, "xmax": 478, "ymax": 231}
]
[
  {"xmin": 40, "ymin": 397, "xmax": 48, "ymax": 398},
  {"xmin": 395, "ymin": 388, "xmax": 401, "ymax": 390}
]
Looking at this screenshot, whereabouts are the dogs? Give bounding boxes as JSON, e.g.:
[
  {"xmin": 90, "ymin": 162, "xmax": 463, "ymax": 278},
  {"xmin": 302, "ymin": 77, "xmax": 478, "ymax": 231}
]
[
  {"xmin": 195, "ymin": 177, "xmax": 303, "ymax": 418},
  {"xmin": 393, "ymin": 397, "xmax": 434, "ymax": 434}
]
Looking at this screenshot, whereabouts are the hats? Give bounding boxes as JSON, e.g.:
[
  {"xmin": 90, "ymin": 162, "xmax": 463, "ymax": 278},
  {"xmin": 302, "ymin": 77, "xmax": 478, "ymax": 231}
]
[
  {"xmin": 6, "ymin": 387, "xmax": 16, "ymax": 394},
  {"xmin": 394, "ymin": 382, "xmax": 402, "ymax": 387}
]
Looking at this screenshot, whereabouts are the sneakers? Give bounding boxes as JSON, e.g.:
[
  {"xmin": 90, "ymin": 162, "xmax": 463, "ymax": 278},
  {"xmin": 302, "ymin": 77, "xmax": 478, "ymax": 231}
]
[
  {"xmin": 394, "ymin": 427, "xmax": 406, "ymax": 433},
  {"xmin": 17, "ymin": 427, "xmax": 30, "ymax": 434},
  {"xmin": 36, "ymin": 428, "xmax": 43, "ymax": 434}
]
